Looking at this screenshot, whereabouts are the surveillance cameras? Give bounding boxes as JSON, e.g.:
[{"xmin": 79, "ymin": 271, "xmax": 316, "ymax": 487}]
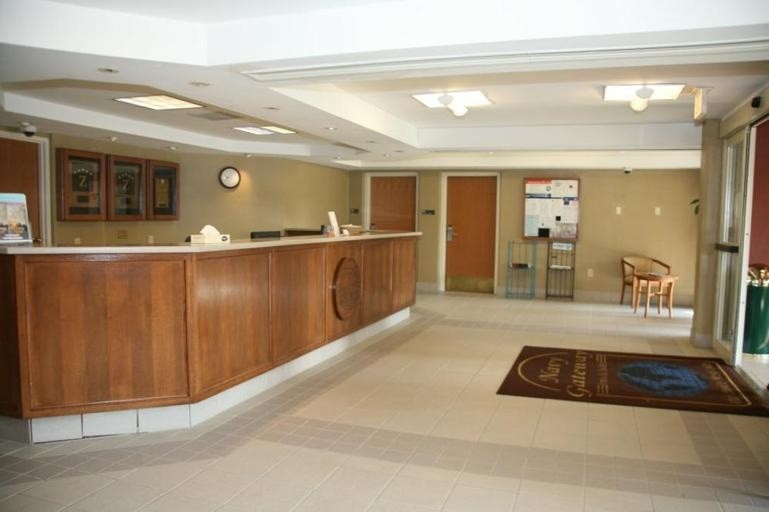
[
  {"xmin": 24, "ymin": 126, "xmax": 37, "ymax": 137},
  {"xmin": 624, "ymin": 169, "xmax": 633, "ymax": 174}
]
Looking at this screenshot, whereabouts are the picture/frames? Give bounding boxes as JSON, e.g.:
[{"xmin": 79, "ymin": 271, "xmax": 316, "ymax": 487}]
[{"xmin": 55, "ymin": 149, "xmax": 180, "ymax": 221}]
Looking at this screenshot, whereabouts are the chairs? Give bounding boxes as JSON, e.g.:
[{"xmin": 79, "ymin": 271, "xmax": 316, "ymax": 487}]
[{"xmin": 620, "ymin": 256, "xmax": 673, "ymax": 313}]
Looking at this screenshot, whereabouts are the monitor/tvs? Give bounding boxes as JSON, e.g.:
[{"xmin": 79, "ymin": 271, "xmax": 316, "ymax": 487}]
[
  {"xmin": 251, "ymin": 230, "xmax": 281, "ymax": 240},
  {"xmin": 285, "ymin": 230, "xmax": 323, "ymax": 236}
]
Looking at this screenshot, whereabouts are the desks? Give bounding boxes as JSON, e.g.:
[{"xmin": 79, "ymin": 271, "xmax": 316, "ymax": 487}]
[{"xmin": 633, "ymin": 273, "xmax": 678, "ymax": 319}]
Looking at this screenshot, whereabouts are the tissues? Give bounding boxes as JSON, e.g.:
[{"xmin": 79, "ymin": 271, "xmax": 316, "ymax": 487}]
[{"xmin": 190, "ymin": 225, "xmax": 231, "ymax": 244}]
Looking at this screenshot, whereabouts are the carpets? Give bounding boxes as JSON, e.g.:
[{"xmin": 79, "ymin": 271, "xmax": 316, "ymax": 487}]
[{"xmin": 496, "ymin": 346, "xmax": 769, "ymax": 419}]
